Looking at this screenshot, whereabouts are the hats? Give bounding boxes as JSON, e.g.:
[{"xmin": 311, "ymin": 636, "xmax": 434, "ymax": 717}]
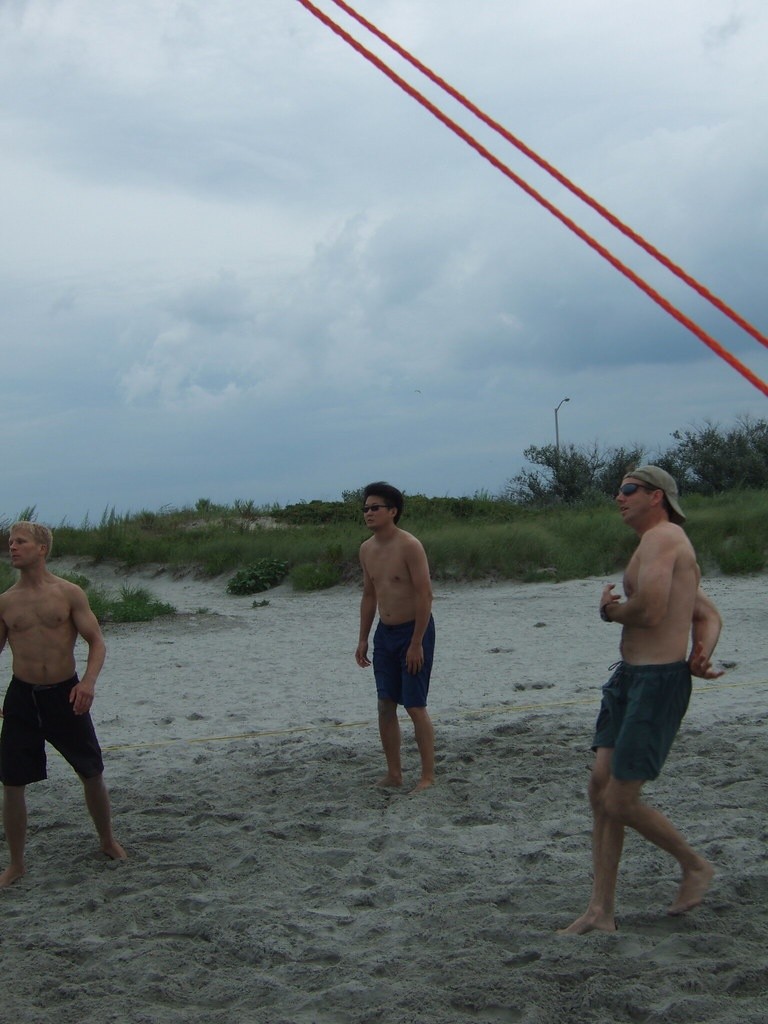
[{"xmin": 622, "ymin": 465, "xmax": 686, "ymax": 525}]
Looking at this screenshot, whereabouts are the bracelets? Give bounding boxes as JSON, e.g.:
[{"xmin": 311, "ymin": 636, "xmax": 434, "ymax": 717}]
[{"xmin": 600, "ymin": 603, "xmax": 612, "ymax": 623}]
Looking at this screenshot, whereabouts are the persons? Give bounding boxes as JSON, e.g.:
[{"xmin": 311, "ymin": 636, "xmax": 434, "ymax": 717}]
[
  {"xmin": 355, "ymin": 482, "xmax": 436, "ymax": 794},
  {"xmin": 558, "ymin": 466, "xmax": 722, "ymax": 935},
  {"xmin": 0, "ymin": 522, "xmax": 126, "ymax": 891}
]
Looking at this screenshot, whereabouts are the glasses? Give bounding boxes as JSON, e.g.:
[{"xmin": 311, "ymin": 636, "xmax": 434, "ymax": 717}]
[
  {"xmin": 615, "ymin": 483, "xmax": 655, "ymax": 496},
  {"xmin": 362, "ymin": 505, "xmax": 392, "ymax": 513}
]
